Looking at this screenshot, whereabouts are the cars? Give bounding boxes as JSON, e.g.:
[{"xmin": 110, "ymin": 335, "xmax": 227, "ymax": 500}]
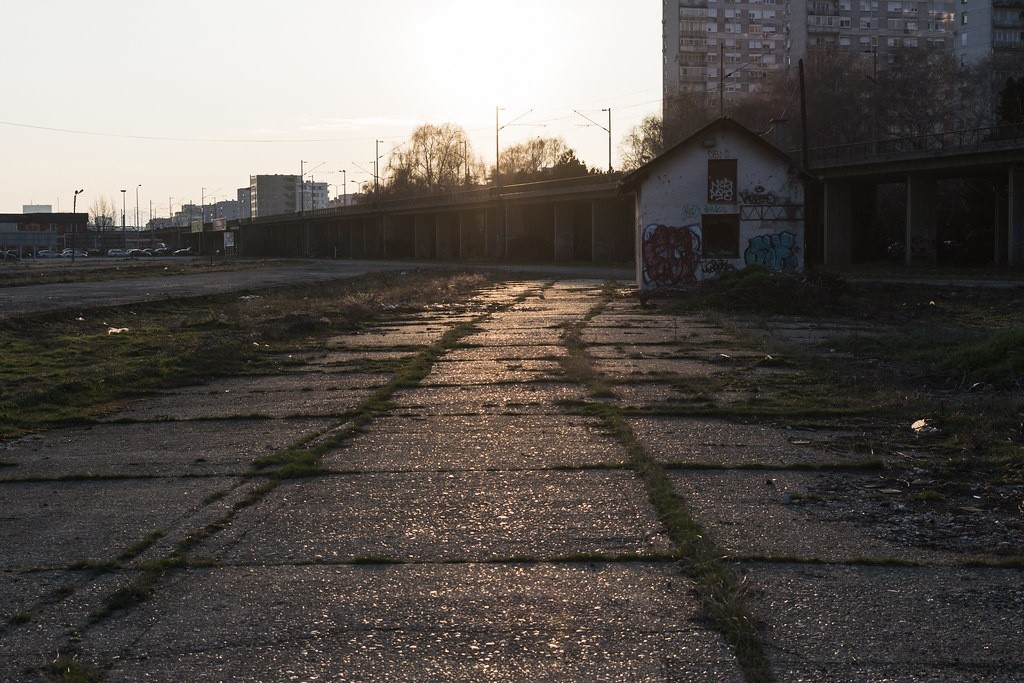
[
  {"xmin": 937, "ymin": 228, "xmax": 994, "ymax": 264},
  {"xmin": 0, "ymin": 247, "xmax": 224, "ymax": 258},
  {"xmin": 882, "ymin": 233, "xmax": 905, "ymax": 261}
]
